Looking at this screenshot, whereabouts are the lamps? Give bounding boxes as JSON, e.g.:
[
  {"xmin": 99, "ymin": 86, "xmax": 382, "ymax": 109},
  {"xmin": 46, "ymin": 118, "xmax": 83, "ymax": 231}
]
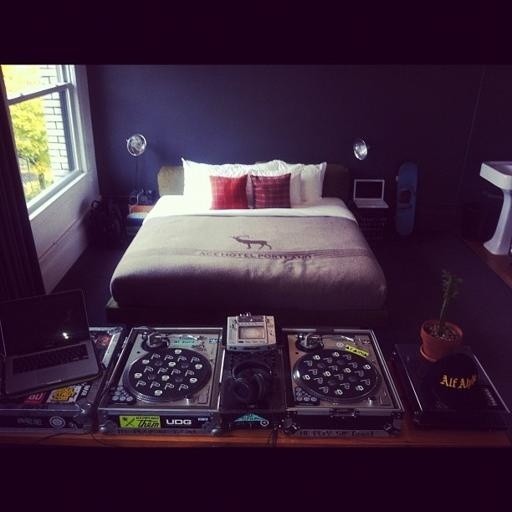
[
  {"xmin": 353, "ymin": 137, "xmax": 377, "ymax": 179},
  {"xmin": 127, "ymin": 134, "xmax": 147, "ymax": 204}
]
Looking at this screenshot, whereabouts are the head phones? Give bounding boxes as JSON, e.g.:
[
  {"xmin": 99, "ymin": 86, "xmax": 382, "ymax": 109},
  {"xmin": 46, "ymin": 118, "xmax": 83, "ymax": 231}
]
[{"xmin": 229, "ymin": 359, "xmax": 274, "ymax": 405}]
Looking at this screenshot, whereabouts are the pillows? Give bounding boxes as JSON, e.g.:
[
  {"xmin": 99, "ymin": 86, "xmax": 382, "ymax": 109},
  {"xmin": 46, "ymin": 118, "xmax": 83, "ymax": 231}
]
[
  {"xmin": 224, "ymin": 163, "xmax": 302, "ymax": 208},
  {"xmin": 180, "ymin": 157, "xmax": 253, "ymax": 211},
  {"xmin": 255, "ymin": 160, "xmax": 328, "ymax": 203},
  {"xmin": 211, "ymin": 174, "xmax": 250, "ymax": 210},
  {"xmin": 249, "ymin": 172, "xmax": 292, "ymax": 208}
]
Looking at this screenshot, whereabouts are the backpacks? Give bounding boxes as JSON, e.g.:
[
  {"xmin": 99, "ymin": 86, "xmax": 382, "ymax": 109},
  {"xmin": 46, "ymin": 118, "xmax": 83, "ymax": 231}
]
[{"xmin": 84, "ymin": 197, "xmax": 128, "ymax": 250}]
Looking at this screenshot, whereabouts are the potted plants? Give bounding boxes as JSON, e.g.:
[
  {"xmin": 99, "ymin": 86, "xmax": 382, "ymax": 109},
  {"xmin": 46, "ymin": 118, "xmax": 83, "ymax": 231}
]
[{"xmin": 420, "ymin": 270, "xmax": 465, "ymax": 366}]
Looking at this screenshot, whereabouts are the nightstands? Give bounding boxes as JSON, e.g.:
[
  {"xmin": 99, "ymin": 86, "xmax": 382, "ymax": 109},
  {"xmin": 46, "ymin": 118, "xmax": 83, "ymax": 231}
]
[
  {"xmin": 129, "ymin": 197, "xmax": 154, "ymax": 212},
  {"xmin": 351, "ymin": 201, "xmax": 390, "ymax": 246}
]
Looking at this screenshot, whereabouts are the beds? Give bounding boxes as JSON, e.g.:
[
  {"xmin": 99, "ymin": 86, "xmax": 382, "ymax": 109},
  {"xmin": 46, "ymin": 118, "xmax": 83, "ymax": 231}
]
[{"xmin": 104, "ymin": 158, "xmax": 391, "ymax": 326}]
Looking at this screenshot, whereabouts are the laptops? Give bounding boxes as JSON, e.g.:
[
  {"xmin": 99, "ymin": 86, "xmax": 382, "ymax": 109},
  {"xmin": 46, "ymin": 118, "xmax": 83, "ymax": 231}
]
[
  {"xmin": 352, "ymin": 179, "xmax": 390, "ymax": 209},
  {"xmin": 0, "ymin": 289, "xmax": 102, "ymax": 402}
]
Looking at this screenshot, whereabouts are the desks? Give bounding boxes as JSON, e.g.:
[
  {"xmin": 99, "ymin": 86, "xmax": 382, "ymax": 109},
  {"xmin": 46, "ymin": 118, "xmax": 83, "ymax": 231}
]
[{"xmin": 0, "ymin": 343, "xmax": 511, "ymax": 449}]
